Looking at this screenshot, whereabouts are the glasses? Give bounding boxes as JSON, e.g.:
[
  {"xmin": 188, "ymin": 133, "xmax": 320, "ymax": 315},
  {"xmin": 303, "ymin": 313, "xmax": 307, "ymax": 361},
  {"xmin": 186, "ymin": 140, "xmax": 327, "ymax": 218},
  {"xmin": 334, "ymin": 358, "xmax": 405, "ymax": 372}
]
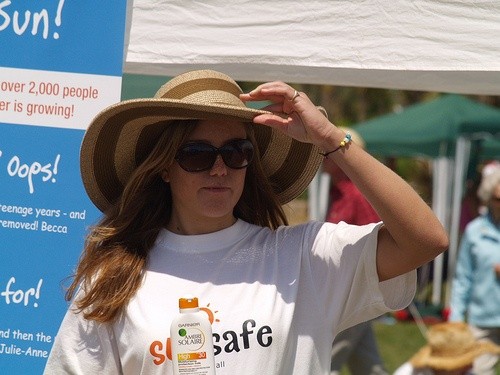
[{"xmin": 174, "ymin": 139, "xmax": 254, "ymax": 173}]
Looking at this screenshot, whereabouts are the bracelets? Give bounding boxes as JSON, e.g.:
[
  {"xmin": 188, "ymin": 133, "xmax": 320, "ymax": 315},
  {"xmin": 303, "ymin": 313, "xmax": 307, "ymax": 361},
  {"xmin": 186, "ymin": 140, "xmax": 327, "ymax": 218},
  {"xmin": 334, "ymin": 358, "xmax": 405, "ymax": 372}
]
[{"xmin": 317, "ymin": 132, "xmax": 352, "ymax": 160}]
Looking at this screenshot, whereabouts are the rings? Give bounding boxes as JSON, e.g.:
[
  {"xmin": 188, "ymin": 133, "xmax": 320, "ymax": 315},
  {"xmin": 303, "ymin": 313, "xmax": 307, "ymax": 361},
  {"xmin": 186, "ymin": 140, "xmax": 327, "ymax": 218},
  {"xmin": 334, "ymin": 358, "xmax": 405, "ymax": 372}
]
[{"xmin": 288, "ymin": 90, "xmax": 300, "ymax": 102}]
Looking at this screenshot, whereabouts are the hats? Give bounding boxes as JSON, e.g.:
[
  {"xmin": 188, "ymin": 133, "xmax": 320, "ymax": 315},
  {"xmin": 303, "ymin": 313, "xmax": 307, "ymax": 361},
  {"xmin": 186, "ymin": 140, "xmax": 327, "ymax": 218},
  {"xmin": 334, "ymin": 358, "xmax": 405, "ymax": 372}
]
[
  {"xmin": 79, "ymin": 71, "xmax": 329, "ymax": 215},
  {"xmin": 411, "ymin": 322, "xmax": 499, "ymax": 370}
]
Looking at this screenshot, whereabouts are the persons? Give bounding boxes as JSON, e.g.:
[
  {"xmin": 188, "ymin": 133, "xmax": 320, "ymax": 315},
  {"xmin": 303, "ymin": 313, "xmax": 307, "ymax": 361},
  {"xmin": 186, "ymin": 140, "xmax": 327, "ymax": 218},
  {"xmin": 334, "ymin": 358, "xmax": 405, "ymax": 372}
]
[
  {"xmin": 325, "ymin": 157, "xmax": 500, "ymax": 375},
  {"xmin": 43, "ymin": 69, "xmax": 451, "ymax": 375}
]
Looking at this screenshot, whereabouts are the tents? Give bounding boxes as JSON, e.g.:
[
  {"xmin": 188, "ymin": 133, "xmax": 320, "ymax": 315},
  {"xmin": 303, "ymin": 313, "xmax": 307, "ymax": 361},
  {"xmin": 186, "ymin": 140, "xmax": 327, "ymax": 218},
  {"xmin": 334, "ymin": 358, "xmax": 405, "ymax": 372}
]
[{"xmin": 121, "ymin": 0, "xmax": 500, "ymax": 308}]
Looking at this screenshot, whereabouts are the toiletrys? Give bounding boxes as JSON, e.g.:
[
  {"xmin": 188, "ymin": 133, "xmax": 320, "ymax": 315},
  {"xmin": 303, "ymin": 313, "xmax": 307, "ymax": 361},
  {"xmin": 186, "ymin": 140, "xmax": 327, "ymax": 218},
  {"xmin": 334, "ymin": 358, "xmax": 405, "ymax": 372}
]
[{"xmin": 170, "ymin": 297, "xmax": 216, "ymax": 375}]
